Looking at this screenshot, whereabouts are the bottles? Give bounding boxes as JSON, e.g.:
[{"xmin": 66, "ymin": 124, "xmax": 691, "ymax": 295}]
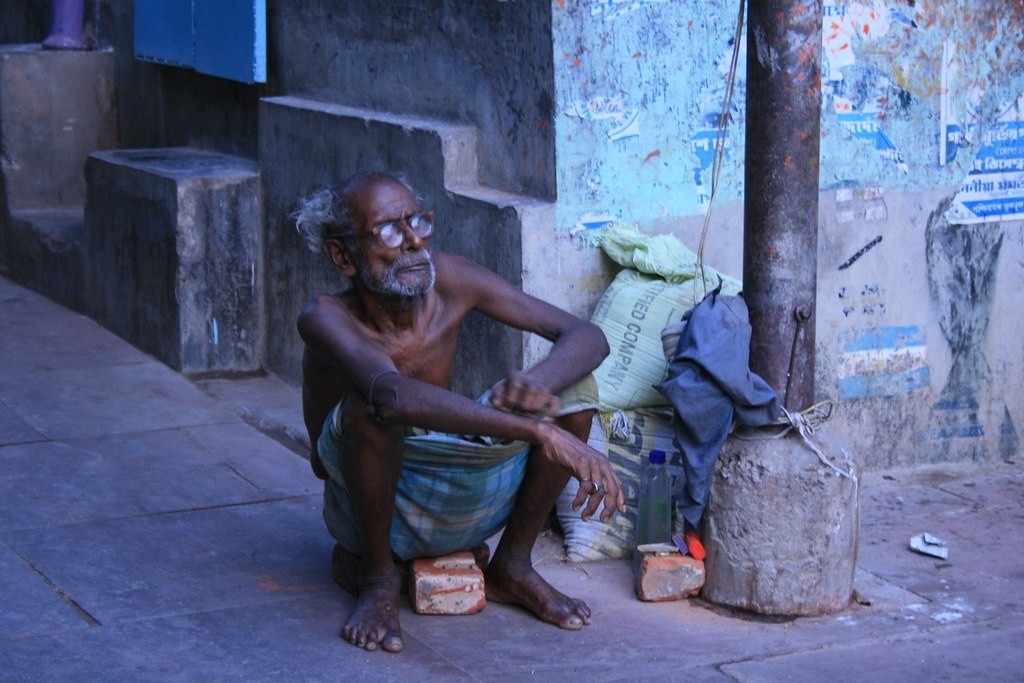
[{"xmin": 631, "ymin": 449, "xmax": 673, "ymax": 574}]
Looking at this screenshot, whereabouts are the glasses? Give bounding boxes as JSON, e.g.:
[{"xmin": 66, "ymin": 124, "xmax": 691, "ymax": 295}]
[{"xmin": 327, "ymin": 210, "xmax": 434, "ymax": 248}]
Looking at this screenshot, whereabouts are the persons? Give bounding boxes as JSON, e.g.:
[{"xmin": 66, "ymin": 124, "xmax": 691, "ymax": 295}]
[{"xmin": 286, "ymin": 168, "xmax": 627, "ymax": 653}]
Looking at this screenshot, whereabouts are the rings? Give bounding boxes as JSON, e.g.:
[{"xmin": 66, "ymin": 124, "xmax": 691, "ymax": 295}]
[
  {"xmin": 579, "ymin": 479, "xmax": 591, "ymax": 482},
  {"xmin": 589, "ymin": 481, "xmax": 604, "ymax": 495}
]
